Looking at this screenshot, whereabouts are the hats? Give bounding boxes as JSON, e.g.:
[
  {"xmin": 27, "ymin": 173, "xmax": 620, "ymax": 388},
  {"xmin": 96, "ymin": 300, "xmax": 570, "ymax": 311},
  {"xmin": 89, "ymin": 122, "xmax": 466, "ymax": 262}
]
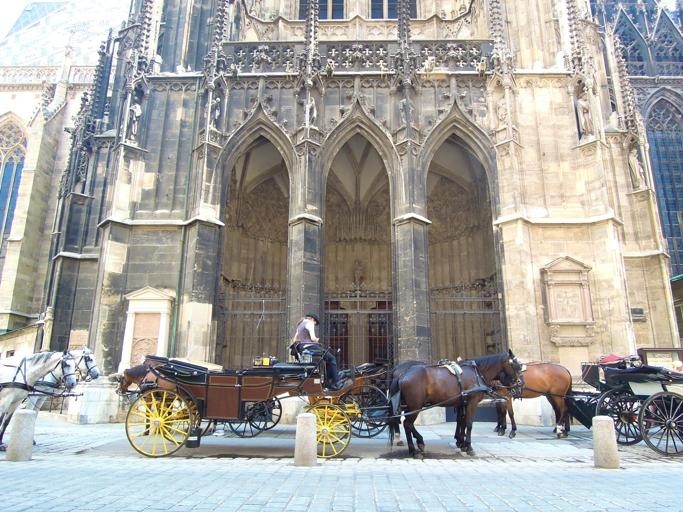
[{"xmin": 305, "ymin": 312, "xmax": 320, "ymax": 326}]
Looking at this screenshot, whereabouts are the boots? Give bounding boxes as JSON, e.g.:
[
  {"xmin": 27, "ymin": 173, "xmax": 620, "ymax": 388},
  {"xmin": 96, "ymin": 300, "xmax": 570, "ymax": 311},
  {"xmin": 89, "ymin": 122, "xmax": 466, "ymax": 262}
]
[{"xmin": 325, "ymin": 359, "xmax": 342, "ymax": 391}]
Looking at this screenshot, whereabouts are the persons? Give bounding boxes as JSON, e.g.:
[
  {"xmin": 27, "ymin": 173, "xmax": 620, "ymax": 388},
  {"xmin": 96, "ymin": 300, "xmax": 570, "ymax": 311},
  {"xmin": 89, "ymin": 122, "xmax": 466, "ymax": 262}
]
[{"xmin": 291, "ymin": 312, "xmax": 339, "ymax": 390}]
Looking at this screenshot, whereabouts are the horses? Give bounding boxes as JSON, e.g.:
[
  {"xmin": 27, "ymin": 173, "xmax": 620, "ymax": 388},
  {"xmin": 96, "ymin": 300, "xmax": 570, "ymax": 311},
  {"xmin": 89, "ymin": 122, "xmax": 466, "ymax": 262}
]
[
  {"xmin": 386, "ymin": 348, "xmax": 573, "ymax": 458},
  {"xmin": 0, "ymin": 346, "xmax": 99, "ymax": 451},
  {"xmin": 118, "ymin": 364, "xmax": 217, "ymax": 435}
]
